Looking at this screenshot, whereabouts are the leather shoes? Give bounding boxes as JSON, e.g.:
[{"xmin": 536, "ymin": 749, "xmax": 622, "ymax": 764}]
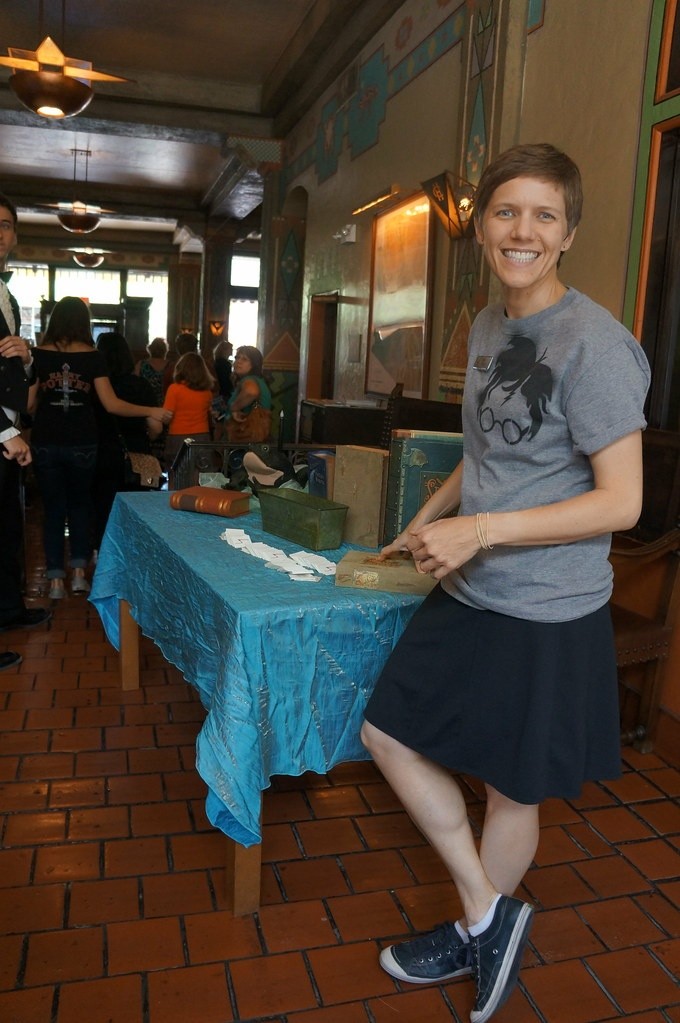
[
  {"xmin": 0, "ymin": 652, "xmax": 22, "ymax": 671},
  {"xmin": 0, "ymin": 608, "xmax": 52, "ymax": 631}
]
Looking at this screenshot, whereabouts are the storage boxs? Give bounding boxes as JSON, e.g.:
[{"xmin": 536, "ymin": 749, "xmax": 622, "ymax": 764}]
[{"xmin": 262, "ymin": 487, "xmax": 348, "ymax": 551}]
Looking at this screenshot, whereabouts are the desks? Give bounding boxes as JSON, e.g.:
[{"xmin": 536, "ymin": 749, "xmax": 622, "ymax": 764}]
[{"xmin": 89, "ymin": 491, "xmax": 430, "ymax": 919}]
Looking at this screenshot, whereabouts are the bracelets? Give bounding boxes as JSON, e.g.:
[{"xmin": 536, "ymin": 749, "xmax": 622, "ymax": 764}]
[{"xmin": 476, "ymin": 512, "xmax": 494, "ymax": 550}]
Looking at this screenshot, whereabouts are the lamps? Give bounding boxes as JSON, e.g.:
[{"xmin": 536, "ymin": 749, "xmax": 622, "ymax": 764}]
[
  {"xmin": 420, "ymin": 170, "xmax": 478, "ymax": 241},
  {"xmin": 39, "ymin": 133, "xmax": 117, "ymax": 234},
  {"xmin": 0, "ymin": 0, "xmax": 136, "ymax": 119},
  {"xmin": 57, "ymin": 246, "xmax": 115, "ymax": 273},
  {"xmin": 333, "ymin": 224, "xmax": 357, "ymax": 244}
]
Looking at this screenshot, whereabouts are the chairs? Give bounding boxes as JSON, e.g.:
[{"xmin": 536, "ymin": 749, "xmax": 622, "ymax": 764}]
[{"xmin": 610, "ymin": 429, "xmax": 679, "ymax": 748}]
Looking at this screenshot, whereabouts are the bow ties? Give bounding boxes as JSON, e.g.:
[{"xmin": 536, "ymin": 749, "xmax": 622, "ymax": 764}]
[{"xmin": 0, "ymin": 271, "xmax": 13, "ymax": 284}]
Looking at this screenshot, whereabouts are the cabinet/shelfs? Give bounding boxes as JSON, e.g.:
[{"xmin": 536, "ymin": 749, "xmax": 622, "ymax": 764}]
[{"xmin": 297, "ymin": 398, "xmax": 325, "ymax": 444}]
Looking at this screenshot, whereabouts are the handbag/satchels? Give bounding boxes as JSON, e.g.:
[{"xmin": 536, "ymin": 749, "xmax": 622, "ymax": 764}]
[
  {"xmin": 124, "ymin": 453, "xmax": 161, "ymax": 490},
  {"xmin": 140, "ymin": 359, "xmax": 163, "ymax": 406},
  {"xmin": 224, "ymin": 378, "xmax": 273, "ymax": 444}
]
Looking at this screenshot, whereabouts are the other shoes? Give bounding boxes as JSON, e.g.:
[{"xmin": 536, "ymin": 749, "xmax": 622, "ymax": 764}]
[
  {"xmin": 48, "ymin": 589, "xmax": 68, "ymax": 598},
  {"xmin": 71, "ymin": 576, "xmax": 91, "ymax": 592}
]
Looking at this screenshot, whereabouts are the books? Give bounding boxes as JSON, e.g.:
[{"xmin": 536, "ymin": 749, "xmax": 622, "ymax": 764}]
[
  {"xmin": 306, "ymin": 450, "xmax": 336, "ymax": 501},
  {"xmin": 334, "ymin": 445, "xmax": 390, "ymax": 549},
  {"xmin": 382, "ymin": 429, "xmax": 464, "ymax": 548},
  {"xmin": 170, "ymin": 486, "xmax": 251, "ymax": 519},
  {"xmin": 336, "ymin": 550, "xmax": 441, "ymax": 597}
]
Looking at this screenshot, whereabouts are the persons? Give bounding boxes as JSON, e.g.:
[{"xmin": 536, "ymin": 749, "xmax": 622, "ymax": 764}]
[
  {"xmin": 0, "ymin": 196, "xmax": 52, "ymax": 668},
  {"xmin": 359, "ymin": 144, "xmax": 651, "ymax": 1023},
  {"xmin": 24, "ymin": 296, "xmax": 273, "ymax": 601}
]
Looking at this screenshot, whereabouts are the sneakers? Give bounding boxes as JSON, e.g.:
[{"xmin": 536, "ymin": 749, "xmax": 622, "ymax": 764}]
[
  {"xmin": 470, "ymin": 896, "xmax": 534, "ymax": 1023},
  {"xmin": 379, "ymin": 923, "xmax": 475, "ymax": 983}
]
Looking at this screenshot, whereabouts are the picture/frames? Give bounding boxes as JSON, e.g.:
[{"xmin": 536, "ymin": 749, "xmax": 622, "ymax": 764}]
[{"xmin": 362, "ymin": 188, "xmax": 432, "ymax": 400}]
